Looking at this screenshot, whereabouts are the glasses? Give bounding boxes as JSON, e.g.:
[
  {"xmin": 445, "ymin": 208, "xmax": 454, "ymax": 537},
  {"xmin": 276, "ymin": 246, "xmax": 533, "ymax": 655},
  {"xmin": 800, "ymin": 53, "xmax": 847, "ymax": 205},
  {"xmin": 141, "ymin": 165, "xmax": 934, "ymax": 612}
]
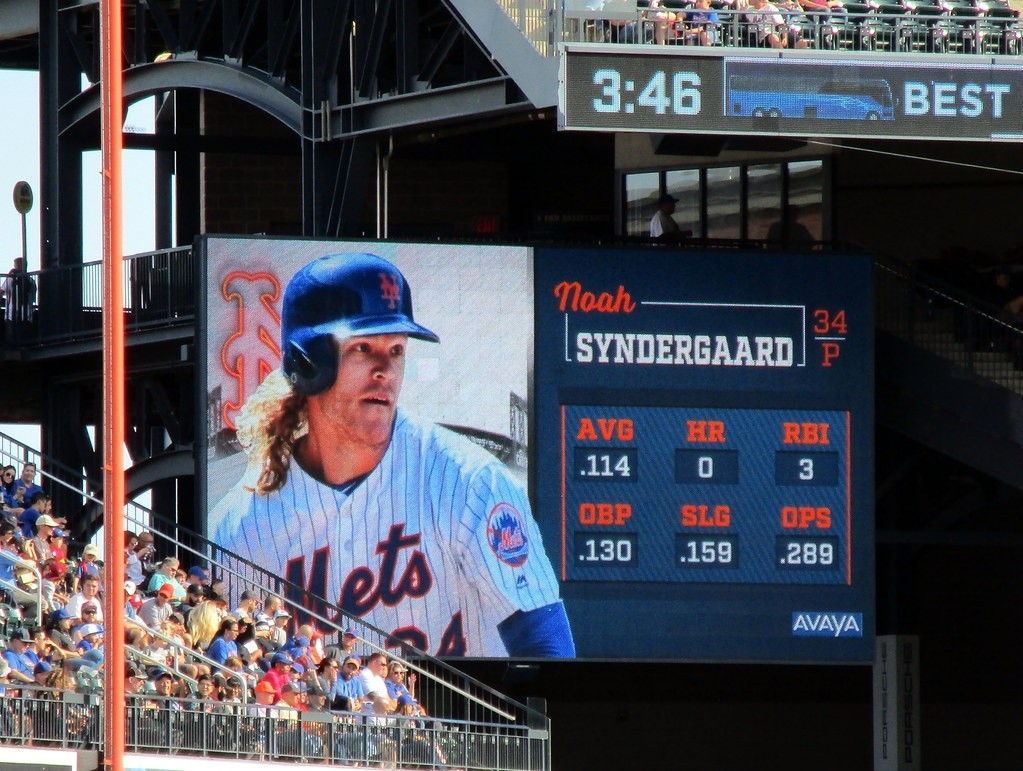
[
  {"xmin": 392, "ymin": 671, "xmax": 404, "ymax": 675},
  {"xmin": 375, "ymin": 660, "xmax": 387, "ymax": 666}
]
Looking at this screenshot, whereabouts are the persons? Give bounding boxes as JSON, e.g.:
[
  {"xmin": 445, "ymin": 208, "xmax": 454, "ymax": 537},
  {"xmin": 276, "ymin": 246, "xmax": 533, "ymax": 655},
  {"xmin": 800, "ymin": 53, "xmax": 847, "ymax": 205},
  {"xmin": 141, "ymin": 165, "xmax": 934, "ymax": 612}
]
[
  {"xmin": 650, "ymin": 195, "xmax": 692, "ymax": 247},
  {"xmin": 603, "ymin": 0, "xmax": 832, "ymax": 48},
  {"xmin": 766, "ymin": 204, "xmax": 813, "ymax": 250},
  {"xmin": 0, "ymin": 258, "xmax": 35, "ymax": 322},
  {"xmin": 0, "ymin": 461, "xmax": 440, "ymax": 770},
  {"xmin": 982, "ymin": 267, "xmax": 1017, "ymax": 306},
  {"xmin": 208, "ymin": 251, "xmax": 576, "ymax": 658}
]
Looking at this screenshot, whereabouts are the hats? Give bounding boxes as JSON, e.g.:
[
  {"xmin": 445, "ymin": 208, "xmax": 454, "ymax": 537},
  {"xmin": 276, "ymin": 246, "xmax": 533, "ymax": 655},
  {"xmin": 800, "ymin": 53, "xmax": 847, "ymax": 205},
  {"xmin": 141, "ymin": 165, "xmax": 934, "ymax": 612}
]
[
  {"xmin": 0, "ymin": 459, "xmax": 154, "ymax": 679},
  {"xmin": 295, "ymin": 682, "xmax": 309, "ymax": 691},
  {"xmin": 398, "ymin": 694, "xmax": 417, "ymax": 705},
  {"xmin": 402, "ymin": 667, "xmax": 408, "ymax": 672},
  {"xmin": 227, "ymin": 677, "xmax": 240, "ymax": 685},
  {"xmin": 344, "ymin": 628, "xmax": 358, "ymax": 638},
  {"xmin": 312, "ymin": 626, "xmax": 323, "ymax": 639},
  {"xmin": 215, "ymin": 676, "xmax": 232, "ymax": 689},
  {"xmin": 255, "ymin": 682, "xmax": 279, "ymax": 693},
  {"xmin": 170, "ymin": 612, "xmax": 184, "ymax": 624},
  {"xmin": 292, "ymin": 663, "xmax": 304, "ymax": 674},
  {"xmin": 156, "ymin": 671, "xmax": 174, "ymax": 682},
  {"xmin": 297, "ymin": 655, "xmax": 319, "ymax": 669},
  {"xmin": 188, "ymin": 584, "xmax": 206, "ymax": 594},
  {"xmin": 271, "ymin": 653, "xmax": 294, "ymax": 664},
  {"xmin": 283, "ymin": 682, "xmax": 305, "ymax": 692},
  {"xmin": 274, "ymin": 610, "xmax": 293, "ymax": 618},
  {"xmin": 298, "ymin": 637, "xmax": 312, "ymax": 648},
  {"xmin": 189, "ymin": 566, "xmax": 209, "ymax": 579},
  {"xmin": 346, "ymin": 655, "xmax": 362, "ymax": 670},
  {"xmin": 241, "ymin": 590, "xmax": 263, "ymax": 603},
  {"xmin": 159, "ymin": 583, "xmax": 174, "ymax": 597},
  {"xmin": 307, "ymin": 686, "xmax": 330, "ymax": 695}
]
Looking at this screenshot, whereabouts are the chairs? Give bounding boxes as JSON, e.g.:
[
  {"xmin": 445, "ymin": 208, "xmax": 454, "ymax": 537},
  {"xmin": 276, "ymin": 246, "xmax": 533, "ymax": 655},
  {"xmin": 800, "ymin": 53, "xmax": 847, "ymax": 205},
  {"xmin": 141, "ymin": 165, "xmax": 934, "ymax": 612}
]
[
  {"xmin": 613, "ymin": 0, "xmax": 1023, "ymax": 55},
  {"xmin": 0, "ymin": 617, "xmax": 36, "ymax": 643}
]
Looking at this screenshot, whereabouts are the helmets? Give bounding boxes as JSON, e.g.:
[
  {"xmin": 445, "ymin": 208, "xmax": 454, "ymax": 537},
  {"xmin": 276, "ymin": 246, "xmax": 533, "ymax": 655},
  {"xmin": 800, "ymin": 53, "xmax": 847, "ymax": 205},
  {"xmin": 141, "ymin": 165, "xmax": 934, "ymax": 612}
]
[{"xmin": 281, "ymin": 251, "xmax": 442, "ymax": 396}]
